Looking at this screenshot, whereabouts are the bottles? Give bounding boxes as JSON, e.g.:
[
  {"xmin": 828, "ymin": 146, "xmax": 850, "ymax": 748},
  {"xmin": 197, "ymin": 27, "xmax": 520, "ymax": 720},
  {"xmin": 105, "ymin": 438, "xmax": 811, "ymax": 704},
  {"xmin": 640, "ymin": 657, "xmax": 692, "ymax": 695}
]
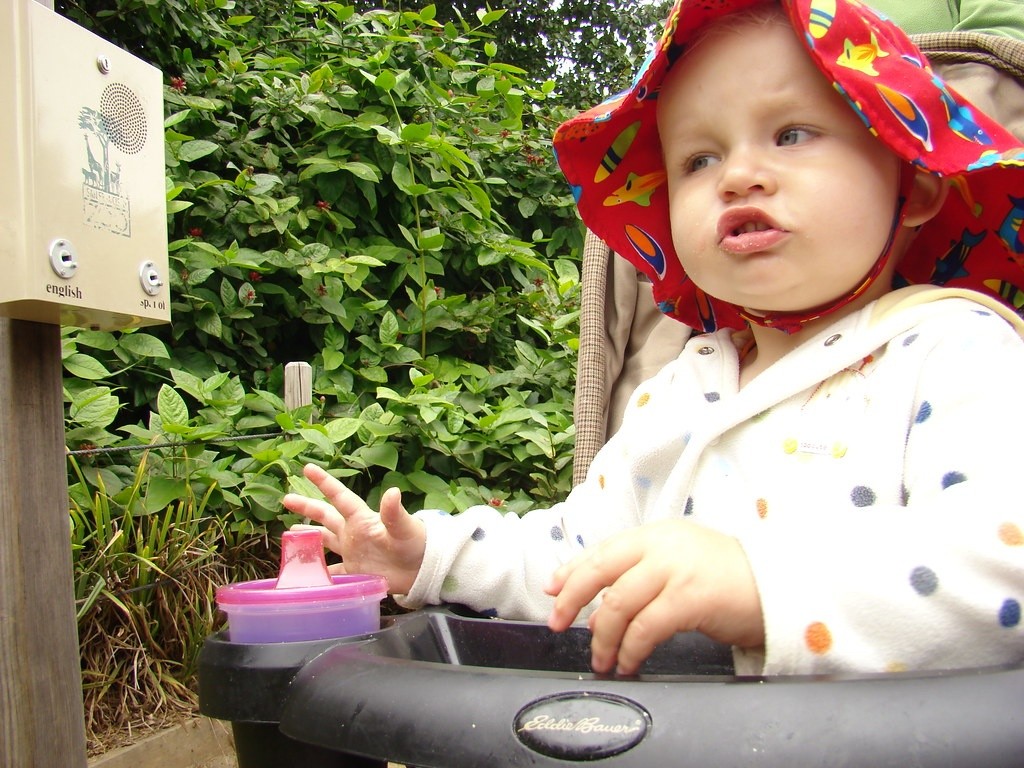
[{"xmin": 215, "ymin": 531, "xmax": 386, "ymax": 643}]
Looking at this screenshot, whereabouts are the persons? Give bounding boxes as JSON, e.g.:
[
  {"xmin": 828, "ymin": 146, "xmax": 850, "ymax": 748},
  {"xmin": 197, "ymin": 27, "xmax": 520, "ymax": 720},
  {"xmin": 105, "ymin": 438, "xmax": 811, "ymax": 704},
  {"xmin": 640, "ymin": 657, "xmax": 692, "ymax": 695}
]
[{"xmin": 281, "ymin": 0, "xmax": 1024, "ymax": 678}]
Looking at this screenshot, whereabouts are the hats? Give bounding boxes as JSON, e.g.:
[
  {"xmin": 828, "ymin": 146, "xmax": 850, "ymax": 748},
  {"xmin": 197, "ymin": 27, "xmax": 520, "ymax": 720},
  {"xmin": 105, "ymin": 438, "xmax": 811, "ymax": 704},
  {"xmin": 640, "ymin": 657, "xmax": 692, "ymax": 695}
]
[{"xmin": 551, "ymin": 0, "xmax": 1024, "ymax": 334}]
